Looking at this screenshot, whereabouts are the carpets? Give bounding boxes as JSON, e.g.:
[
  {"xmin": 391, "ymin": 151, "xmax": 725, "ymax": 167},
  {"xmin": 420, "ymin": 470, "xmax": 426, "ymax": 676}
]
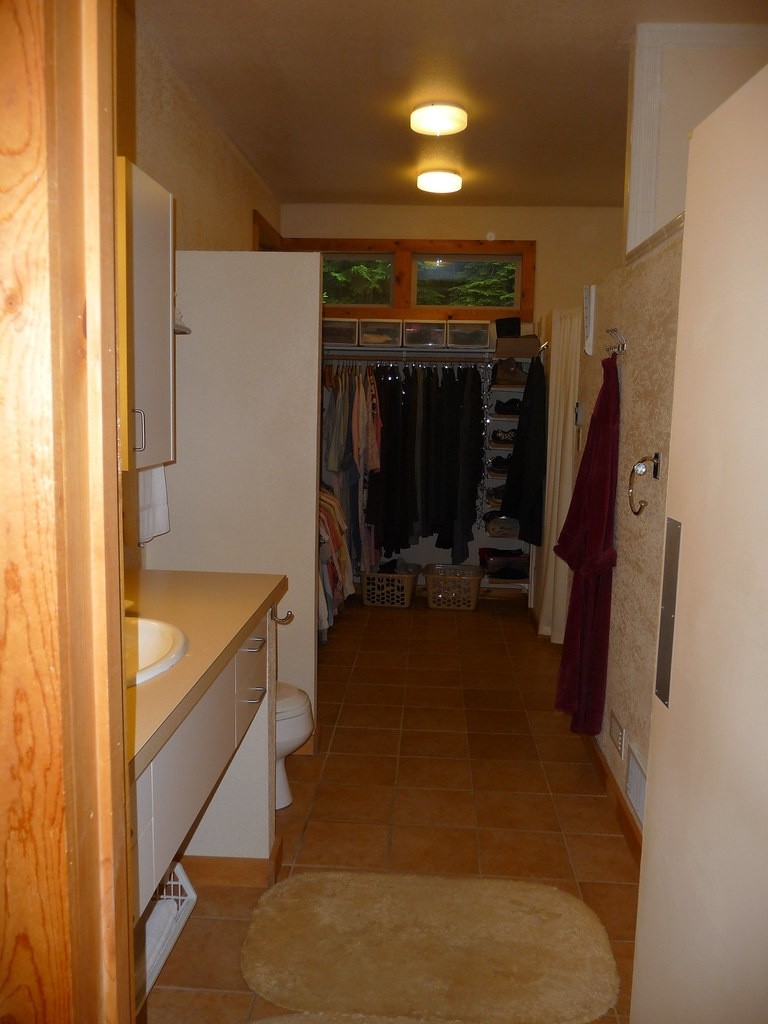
[{"xmin": 239, "ymin": 871, "xmax": 622, "ymax": 1024}]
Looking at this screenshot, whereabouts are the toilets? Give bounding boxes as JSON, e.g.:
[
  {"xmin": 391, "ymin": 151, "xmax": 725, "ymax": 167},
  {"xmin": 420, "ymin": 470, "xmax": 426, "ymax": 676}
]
[{"xmin": 275, "ymin": 681, "xmax": 315, "ymax": 811}]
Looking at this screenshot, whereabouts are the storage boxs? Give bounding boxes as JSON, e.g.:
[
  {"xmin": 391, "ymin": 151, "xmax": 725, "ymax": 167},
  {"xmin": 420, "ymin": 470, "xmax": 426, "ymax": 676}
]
[
  {"xmin": 403, "ymin": 318, "xmax": 446, "ymax": 349},
  {"xmin": 322, "ymin": 316, "xmax": 357, "ymax": 347},
  {"xmin": 420, "ymin": 561, "xmax": 488, "ymax": 613},
  {"xmin": 448, "ymin": 318, "xmax": 492, "ymax": 350},
  {"xmin": 357, "ymin": 556, "xmax": 423, "ymax": 611},
  {"xmin": 359, "ymin": 317, "xmax": 403, "ymax": 348}
]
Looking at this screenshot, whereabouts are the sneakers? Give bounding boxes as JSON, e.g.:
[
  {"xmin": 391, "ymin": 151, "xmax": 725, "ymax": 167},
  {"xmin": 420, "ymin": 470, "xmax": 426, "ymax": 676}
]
[{"xmin": 495, "ymin": 366, "xmax": 527, "ymax": 385}]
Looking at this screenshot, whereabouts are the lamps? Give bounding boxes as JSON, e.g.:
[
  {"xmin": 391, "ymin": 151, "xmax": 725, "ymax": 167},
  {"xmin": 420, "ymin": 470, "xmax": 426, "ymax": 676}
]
[
  {"xmin": 414, "ymin": 166, "xmax": 465, "ymax": 197},
  {"xmin": 408, "ymin": 99, "xmax": 468, "ymax": 139}
]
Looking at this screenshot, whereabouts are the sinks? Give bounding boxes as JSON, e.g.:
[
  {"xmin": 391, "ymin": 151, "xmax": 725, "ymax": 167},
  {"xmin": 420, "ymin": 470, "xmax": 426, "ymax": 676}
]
[{"xmin": 137, "ymin": 617, "xmax": 187, "ymax": 686}]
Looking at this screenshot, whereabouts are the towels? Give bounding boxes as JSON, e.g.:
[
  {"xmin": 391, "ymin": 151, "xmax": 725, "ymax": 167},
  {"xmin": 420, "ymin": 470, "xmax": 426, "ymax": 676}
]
[
  {"xmin": 138, "ymin": 462, "xmax": 170, "ymax": 543},
  {"xmin": 133, "ymin": 899, "xmax": 178, "ymax": 997}
]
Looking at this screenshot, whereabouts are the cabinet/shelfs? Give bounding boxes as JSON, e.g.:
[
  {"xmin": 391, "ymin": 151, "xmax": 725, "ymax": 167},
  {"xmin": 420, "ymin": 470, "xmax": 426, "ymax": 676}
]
[
  {"xmin": 132, "ymin": 605, "xmax": 280, "ymax": 1019},
  {"xmin": 478, "ymin": 335, "xmax": 539, "ymax": 591},
  {"xmin": 115, "ymin": 150, "xmax": 178, "ymax": 478}
]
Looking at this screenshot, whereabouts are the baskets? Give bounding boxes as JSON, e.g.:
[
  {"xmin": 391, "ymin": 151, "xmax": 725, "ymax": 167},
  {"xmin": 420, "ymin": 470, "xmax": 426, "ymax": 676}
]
[
  {"xmin": 422, "ymin": 564, "xmax": 483, "ymax": 610},
  {"xmin": 359, "ymin": 569, "xmax": 418, "ymax": 608}
]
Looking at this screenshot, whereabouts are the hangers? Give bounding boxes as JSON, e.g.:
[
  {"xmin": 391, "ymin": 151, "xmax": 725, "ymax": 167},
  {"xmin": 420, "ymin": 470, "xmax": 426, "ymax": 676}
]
[{"xmin": 323, "ymin": 354, "xmax": 489, "ymax": 370}]
